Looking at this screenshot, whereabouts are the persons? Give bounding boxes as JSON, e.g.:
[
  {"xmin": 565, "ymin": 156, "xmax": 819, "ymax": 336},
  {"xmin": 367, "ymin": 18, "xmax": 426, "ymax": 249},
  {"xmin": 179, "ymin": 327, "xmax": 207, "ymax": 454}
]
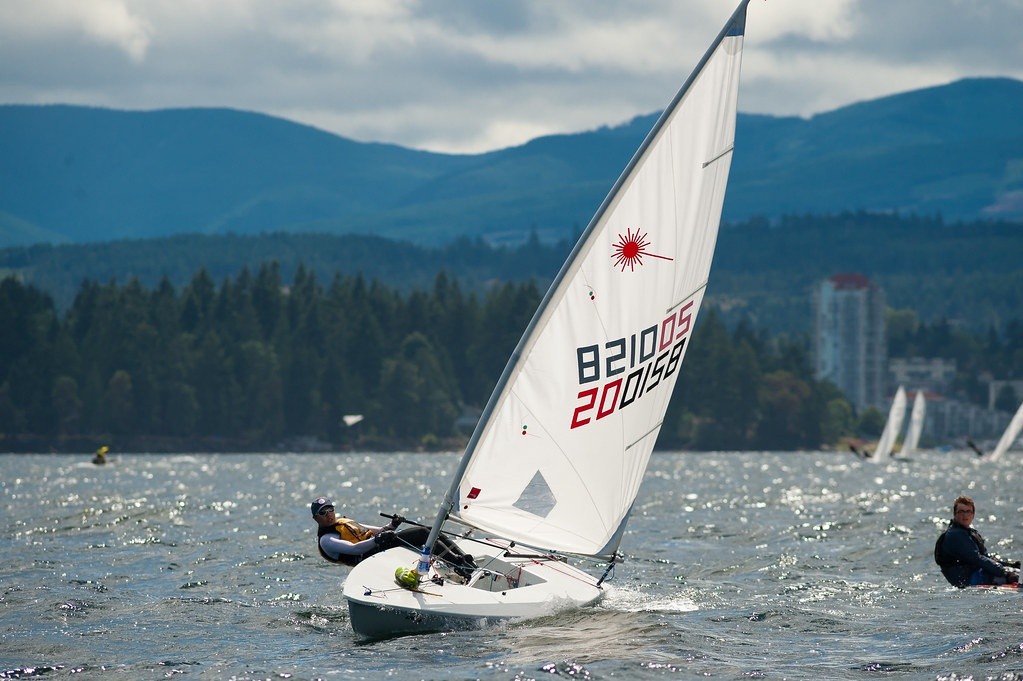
[
  {"xmin": 92, "ymin": 449, "xmax": 106, "ymax": 465},
  {"xmin": 934, "ymin": 497, "xmax": 1023, "ymax": 589},
  {"xmin": 311, "ymin": 497, "xmax": 474, "ymax": 565}
]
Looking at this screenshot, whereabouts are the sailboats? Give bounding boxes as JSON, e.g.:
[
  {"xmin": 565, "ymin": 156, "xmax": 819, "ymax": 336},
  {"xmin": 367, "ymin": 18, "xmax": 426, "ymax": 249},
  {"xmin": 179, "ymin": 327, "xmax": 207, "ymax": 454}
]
[
  {"xmin": 848, "ymin": 384, "xmax": 926, "ymax": 467},
  {"xmin": 338, "ymin": 0, "xmax": 747, "ymax": 635},
  {"xmin": 967, "ymin": 402, "xmax": 1023, "ymax": 469}
]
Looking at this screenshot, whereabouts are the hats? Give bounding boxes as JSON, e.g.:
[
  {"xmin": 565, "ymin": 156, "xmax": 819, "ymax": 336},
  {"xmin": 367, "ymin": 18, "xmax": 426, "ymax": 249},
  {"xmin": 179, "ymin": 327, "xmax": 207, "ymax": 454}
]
[{"xmin": 311, "ymin": 497, "xmax": 333, "ymax": 516}]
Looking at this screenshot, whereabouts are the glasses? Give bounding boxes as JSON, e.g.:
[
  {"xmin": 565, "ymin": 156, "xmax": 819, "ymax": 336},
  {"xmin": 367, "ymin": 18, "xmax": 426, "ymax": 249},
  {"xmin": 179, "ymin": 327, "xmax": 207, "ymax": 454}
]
[
  {"xmin": 315, "ymin": 506, "xmax": 334, "ymax": 517},
  {"xmin": 955, "ymin": 510, "xmax": 974, "ymax": 516}
]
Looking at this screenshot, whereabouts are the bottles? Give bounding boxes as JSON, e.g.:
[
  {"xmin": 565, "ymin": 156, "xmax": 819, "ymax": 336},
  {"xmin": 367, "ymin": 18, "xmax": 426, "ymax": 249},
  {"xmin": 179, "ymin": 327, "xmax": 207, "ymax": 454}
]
[{"xmin": 417, "ymin": 546, "xmax": 431, "ymax": 576}]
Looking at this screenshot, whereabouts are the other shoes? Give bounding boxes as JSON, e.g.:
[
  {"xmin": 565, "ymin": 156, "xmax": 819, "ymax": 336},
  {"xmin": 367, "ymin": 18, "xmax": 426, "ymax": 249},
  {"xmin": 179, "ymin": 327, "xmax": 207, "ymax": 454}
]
[{"xmin": 453, "ymin": 554, "xmax": 491, "ymax": 580}]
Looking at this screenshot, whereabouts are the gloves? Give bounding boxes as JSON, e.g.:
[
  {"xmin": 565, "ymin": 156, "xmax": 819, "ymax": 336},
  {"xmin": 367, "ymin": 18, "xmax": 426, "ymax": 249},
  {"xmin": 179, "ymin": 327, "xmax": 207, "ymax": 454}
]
[
  {"xmin": 392, "ymin": 514, "xmax": 406, "ymax": 527},
  {"xmin": 375, "ymin": 530, "xmax": 397, "ymax": 543},
  {"xmin": 1005, "ymin": 570, "xmax": 1020, "ymax": 584}
]
